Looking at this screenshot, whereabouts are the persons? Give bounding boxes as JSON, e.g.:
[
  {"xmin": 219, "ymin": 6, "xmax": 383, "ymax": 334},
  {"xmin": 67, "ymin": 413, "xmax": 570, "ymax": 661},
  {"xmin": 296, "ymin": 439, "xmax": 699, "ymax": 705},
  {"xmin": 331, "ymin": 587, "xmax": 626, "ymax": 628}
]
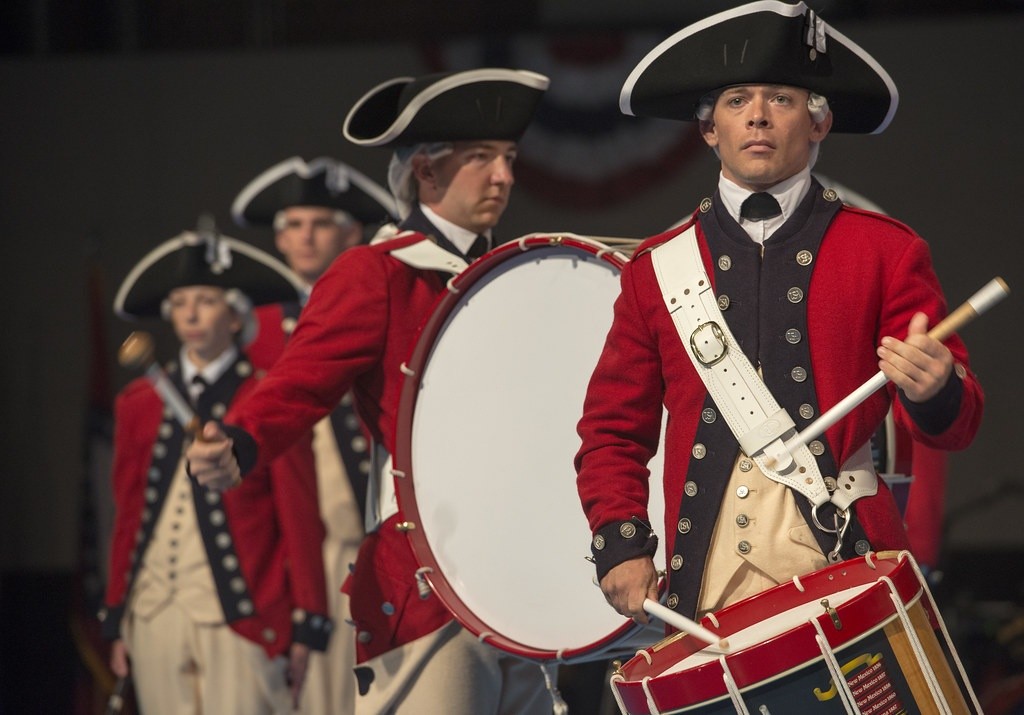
[{"xmin": 92, "ymin": 0, "xmax": 992, "ymax": 715}]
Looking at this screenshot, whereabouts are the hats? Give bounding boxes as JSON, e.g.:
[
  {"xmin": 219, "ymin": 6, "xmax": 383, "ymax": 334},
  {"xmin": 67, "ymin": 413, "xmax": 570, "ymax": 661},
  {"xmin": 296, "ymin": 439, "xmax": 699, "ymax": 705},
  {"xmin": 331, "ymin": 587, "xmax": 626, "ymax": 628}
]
[
  {"xmin": 342, "ymin": 68, "xmax": 550, "ymax": 145},
  {"xmin": 113, "ymin": 231, "xmax": 307, "ymax": 331},
  {"xmin": 231, "ymin": 155, "xmax": 401, "ymax": 229},
  {"xmin": 619, "ymin": 0, "xmax": 900, "ymax": 136}
]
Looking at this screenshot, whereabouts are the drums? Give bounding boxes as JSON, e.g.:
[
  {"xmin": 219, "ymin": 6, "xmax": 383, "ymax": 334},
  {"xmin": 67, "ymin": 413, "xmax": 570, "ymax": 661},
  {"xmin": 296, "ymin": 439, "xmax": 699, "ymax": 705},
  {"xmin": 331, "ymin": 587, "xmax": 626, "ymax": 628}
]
[{"xmin": 393, "ymin": 232, "xmax": 980, "ymax": 714}]
